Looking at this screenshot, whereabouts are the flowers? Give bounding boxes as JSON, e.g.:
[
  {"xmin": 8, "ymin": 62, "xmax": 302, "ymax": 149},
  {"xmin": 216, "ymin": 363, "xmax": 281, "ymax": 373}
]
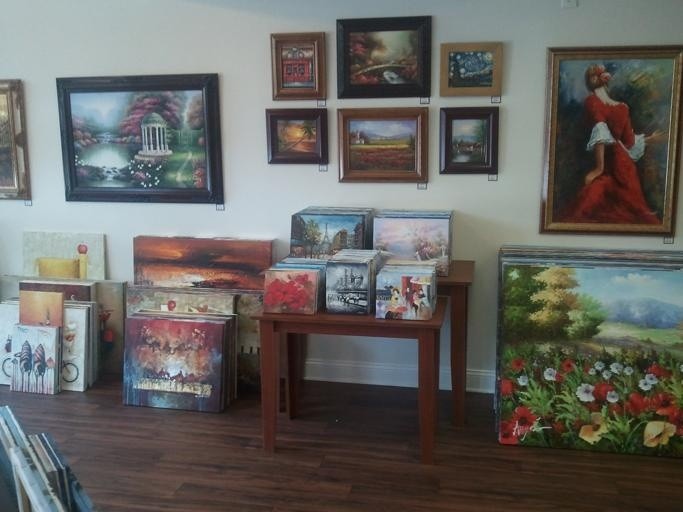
[{"xmin": 498, "ymin": 343, "xmax": 682, "ymax": 454}]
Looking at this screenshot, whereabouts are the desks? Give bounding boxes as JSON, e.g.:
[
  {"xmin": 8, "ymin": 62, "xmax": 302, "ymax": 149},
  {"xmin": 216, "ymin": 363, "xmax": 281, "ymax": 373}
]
[
  {"xmin": 250, "ymin": 296, "xmax": 446, "ymax": 466},
  {"xmin": 439, "ymin": 260, "xmax": 475, "ymax": 425}
]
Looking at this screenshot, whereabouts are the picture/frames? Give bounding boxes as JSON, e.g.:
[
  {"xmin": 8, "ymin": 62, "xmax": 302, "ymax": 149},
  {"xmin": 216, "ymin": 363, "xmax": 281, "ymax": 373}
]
[
  {"xmin": 538, "ymin": 44, "xmax": 682, "ymax": 238},
  {"xmin": 56, "ymin": 73, "xmax": 224, "ymax": 204},
  {"xmin": 440, "ymin": 107, "xmax": 498, "ymax": 175},
  {"xmin": 266, "ymin": 108, "xmax": 329, "ymax": 165},
  {"xmin": 335, "ymin": 16, "xmax": 431, "ymax": 99},
  {"xmin": 336, "ymin": 106, "xmax": 429, "ymax": 183},
  {"xmin": 270, "ymin": 32, "xmax": 326, "ymax": 101},
  {"xmin": 439, "ymin": 41, "xmax": 501, "ymax": 97},
  {"xmin": 0, "ymin": 79, "xmax": 30, "ymax": 200}
]
